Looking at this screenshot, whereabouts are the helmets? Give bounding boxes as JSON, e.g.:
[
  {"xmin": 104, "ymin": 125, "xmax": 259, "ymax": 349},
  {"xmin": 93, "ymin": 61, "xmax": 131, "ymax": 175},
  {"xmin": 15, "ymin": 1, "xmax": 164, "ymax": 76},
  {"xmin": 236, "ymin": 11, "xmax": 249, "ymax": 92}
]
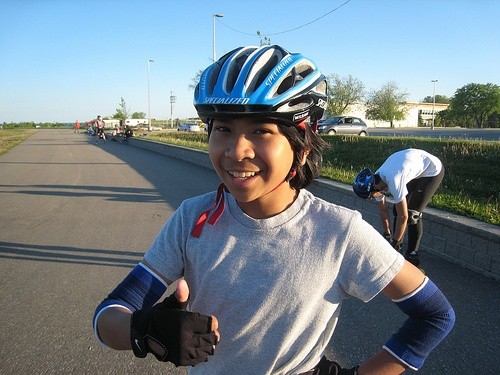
[
  {"xmin": 192, "ymin": 44, "xmax": 329, "ymax": 123},
  {"xmin": 352, "ymin": 167, "xmax": 374, "ymax": 199}
]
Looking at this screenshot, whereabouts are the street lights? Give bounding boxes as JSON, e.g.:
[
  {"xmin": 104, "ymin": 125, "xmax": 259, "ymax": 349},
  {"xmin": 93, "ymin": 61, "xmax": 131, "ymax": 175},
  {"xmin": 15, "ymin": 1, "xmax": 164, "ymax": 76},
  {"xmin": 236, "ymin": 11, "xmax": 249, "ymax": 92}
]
[
  {"xmin": 431, "ymin": 79, "xmax": 439, "ymax": 131},
  {"xmin": 147, "ymin": 59, "xmax": 155, "ymax": 130},
  {"xmin": 212, "ymin": 12, "xmax": 224, "ymax": 63}
]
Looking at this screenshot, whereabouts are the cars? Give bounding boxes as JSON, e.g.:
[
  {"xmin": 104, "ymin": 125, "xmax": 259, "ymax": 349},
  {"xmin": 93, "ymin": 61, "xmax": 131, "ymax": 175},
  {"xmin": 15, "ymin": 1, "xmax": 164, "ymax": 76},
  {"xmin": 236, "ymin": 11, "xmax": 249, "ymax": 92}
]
[
  {"xmin": 311, "ymin": 116, "xmax": 369, "ymax": 137},
  {"xmin": 176, "ymin": 122, "xmax": 201, "ymax": 133},
  {"xmin": 309, "ymin": 120, "xmax": 325, "ymax": 127}
]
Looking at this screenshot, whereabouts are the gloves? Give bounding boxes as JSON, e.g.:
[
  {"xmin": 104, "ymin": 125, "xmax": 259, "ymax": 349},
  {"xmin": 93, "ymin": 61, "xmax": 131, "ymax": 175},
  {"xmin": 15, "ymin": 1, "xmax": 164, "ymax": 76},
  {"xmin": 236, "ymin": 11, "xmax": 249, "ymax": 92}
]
[
  {"xmin": 382, "ymin": 229, "xmax": 393, "ymax": 245},
  {"xmin": 392, "ymin": 236, "xmax": 404, "ymax": 254}
]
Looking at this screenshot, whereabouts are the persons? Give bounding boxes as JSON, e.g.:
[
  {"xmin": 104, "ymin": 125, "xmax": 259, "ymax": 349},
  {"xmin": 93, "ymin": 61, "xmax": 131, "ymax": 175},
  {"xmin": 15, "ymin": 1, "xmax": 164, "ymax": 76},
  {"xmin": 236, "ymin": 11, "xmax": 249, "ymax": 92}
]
[
  {"xmin": 73, "ymin": 115, "xmax": 132, "ymax": 144},
  {"xmin": 93, "ymin": 46, "xmax": 455, "ymax": 375},
  {"xmin": 352, "ymin": 149, "xmax": 446, "ymax": 268}
]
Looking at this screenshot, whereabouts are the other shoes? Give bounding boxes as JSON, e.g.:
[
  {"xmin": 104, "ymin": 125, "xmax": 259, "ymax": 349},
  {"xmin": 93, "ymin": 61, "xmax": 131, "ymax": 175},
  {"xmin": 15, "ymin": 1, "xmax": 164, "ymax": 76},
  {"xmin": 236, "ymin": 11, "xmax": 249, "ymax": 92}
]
[{"xmin": 404, "ymin": 249, "xmax": 420, "ymax": 267}]
